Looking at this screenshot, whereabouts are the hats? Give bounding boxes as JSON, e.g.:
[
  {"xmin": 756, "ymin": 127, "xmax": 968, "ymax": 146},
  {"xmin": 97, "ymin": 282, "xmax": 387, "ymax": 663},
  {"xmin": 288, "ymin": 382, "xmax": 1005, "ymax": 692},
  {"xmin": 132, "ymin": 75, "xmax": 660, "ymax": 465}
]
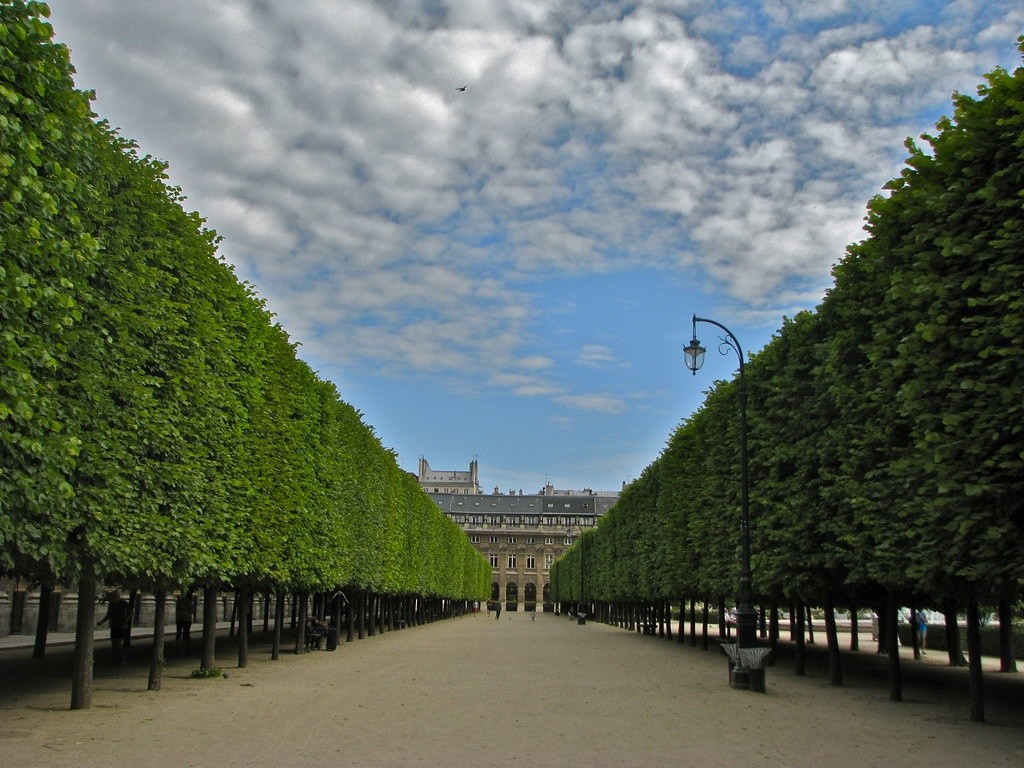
[{"xmin": 100, "ymin": 586, "xmax": 120, "ymax": 604}]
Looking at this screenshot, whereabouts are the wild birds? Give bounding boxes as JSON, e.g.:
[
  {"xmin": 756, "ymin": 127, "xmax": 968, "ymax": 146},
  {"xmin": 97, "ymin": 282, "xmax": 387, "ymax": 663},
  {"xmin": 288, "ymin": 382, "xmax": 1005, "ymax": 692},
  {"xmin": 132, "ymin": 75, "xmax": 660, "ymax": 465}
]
[{"xmin": 455, "ymin": 85, "xmax": 467, "ymax": 92}]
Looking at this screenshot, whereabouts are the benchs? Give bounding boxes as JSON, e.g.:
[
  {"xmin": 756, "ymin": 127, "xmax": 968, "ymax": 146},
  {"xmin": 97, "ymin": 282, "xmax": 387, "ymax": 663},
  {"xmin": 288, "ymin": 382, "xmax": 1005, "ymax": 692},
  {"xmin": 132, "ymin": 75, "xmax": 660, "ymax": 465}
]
[
  {"xmin": 292, "ymin": 621, "xmax": 328, "ymax": 652},
  {"xmin": 640, "ymin": 624, "xmax": 657, "ymax": 635}
]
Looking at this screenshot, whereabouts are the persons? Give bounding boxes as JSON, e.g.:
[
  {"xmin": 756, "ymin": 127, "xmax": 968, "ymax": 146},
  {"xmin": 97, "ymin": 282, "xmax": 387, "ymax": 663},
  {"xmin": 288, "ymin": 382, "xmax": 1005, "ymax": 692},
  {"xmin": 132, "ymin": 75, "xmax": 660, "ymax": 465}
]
[
  {"xmin": 495, "ymin": 601, "xmax": 502, "ymax": 620},
  {"xmin": 472, "ymin": 599, "xmax": 479, "ymax": 616},
  {"xmin": 871, "ymin": 612, "xmax": 878, "ymax": 640},
  {"xmin": 486, "ymin": 598, "xmax": 490, "ymax": 613},
  {"xmin": 916, "ymin": 609, "xmax": 927, "ymax": 654},
  {"xmin": 97, "ymin": 591, "xmax": 133, "ymax": 667},
  {"xmin": 726, "ymin": 612, "xmax": 736, "ymax": 629},
  {"xmin": 312, "ymin": 618, "xmax": 327, "ymax": 649},
  {"xmin": 568, "ymin": 609, "xmax": 572, "ymax": 621},
  {"xmin": 175, "ymin": 593, "xmax": 195, "ymax": 642}
]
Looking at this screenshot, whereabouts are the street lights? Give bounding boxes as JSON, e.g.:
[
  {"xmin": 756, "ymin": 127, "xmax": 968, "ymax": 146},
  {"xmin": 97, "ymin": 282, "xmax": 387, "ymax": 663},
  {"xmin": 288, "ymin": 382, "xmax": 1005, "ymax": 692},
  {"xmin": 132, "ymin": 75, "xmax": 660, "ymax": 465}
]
[
  {"xmin": 548, "ymin": 558, "xmax": 559, "ymax": 615},
  {"xmin": 566, "ymin": 522, "xmax": 587, "ymax": 625},
  {"xmin": 682, "ymin": 313, "xmax": 772, "ymax": 692}
]
